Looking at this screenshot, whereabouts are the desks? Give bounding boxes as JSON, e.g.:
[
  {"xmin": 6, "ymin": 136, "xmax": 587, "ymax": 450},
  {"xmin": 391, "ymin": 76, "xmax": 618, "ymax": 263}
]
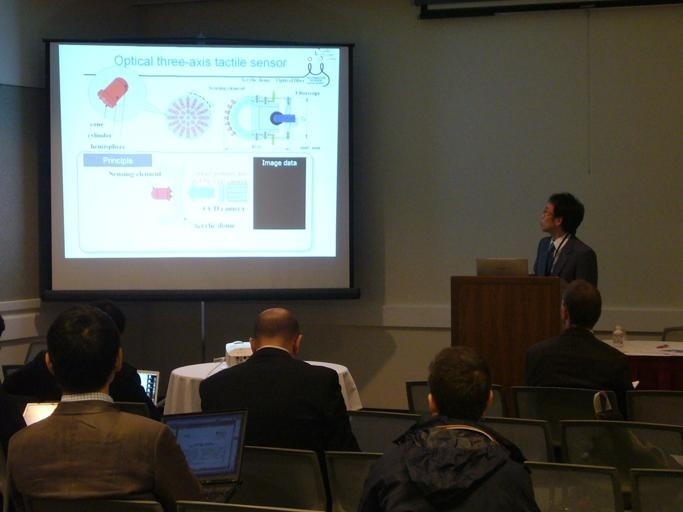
[
  {"xmin": 163, "ymin": 360, "xmax": 362, "ymax": 415},
  {"xmin": 597, "ymin": 339, "xmax": 682, "ymax": 388}
]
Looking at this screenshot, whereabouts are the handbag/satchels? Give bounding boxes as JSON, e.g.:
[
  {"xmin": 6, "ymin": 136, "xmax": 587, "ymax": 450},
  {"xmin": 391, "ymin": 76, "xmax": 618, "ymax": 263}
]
[{"xmin": 592, "ymin": 391, "xmax": 681, "ymax": 469}]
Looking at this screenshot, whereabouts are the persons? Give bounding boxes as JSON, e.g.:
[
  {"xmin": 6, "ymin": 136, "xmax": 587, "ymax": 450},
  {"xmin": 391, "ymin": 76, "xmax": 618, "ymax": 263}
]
[
  {"xmin": 354, "ymin": 345, "xmax": 542, "ymax": 512},
  {"xmin": 531, "ymin": 190, "xmax": 600, "ymax": 291},
  {"xmin": 4, "ymin": 299, "xmax": 163, "ymax": 423},
  {"xmin": 198, "ymin": 306, "xmax": 361, "ymax": 457},
  {"xmin": 8, "ymin": 304, "xmax": 205, "ymax": 511},
  {"xmin": 522, "ymin": 275, "xmax": 636, "ymax": 427}
]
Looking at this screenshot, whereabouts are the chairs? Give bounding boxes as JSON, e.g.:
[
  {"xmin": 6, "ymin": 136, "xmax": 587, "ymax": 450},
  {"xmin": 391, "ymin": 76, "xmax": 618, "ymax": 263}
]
[{"xmin": 663, "ymin": 327, "xmax": 683, "ymax": 341}]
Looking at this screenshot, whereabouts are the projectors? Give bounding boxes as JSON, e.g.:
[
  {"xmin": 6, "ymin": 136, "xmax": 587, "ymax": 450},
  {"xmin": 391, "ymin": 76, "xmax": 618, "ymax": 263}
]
[{"xmin": 224, "ymin": 341, "xmax": 253, "ymax": 368}]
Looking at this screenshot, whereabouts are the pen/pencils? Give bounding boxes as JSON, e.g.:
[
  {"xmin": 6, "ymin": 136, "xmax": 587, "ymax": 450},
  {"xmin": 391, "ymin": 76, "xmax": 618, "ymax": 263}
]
[{"xmin": 656, "ymin": 344, "xmax": 669, "ymax": 348}]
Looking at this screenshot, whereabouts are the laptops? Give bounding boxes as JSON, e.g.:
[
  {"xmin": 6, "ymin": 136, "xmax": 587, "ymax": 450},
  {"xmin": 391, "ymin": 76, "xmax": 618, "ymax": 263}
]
[
  {"xmin": 136, "ymin": 369, "xmax": 160, "ymax": 407},
  {"xmin": 160, "ymin": 408, "xmax": 250, "ymax": 503},
  {"xmin": 19, "ymin": 399, "xmax": 59, "ymax": 427}
]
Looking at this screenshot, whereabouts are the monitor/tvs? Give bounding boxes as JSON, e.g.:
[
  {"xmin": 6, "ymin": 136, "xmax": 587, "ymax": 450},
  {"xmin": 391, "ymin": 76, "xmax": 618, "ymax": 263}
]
[{"xmin": 475, "ymin": 258, "xmax": 529, "ymax": 277}]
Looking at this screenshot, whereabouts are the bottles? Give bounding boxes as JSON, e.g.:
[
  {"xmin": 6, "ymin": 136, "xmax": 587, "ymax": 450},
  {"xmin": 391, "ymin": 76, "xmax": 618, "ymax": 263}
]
[{"xmin": 612, "ymin": 325, "xmax": 626, "ymax": 347}]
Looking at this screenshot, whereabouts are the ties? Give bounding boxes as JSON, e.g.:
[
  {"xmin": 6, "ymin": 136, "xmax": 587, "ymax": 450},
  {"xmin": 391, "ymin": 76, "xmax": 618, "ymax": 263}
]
[{"xmin": 546, "ymin": 243, "xmax": 555, "ymax": 274}]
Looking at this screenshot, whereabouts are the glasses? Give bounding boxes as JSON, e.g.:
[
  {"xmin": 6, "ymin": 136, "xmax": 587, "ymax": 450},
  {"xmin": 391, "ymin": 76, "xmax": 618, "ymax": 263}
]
[{"xmin": 544, "ymin": 207, "xmax": 554, "ymax": 219}]
